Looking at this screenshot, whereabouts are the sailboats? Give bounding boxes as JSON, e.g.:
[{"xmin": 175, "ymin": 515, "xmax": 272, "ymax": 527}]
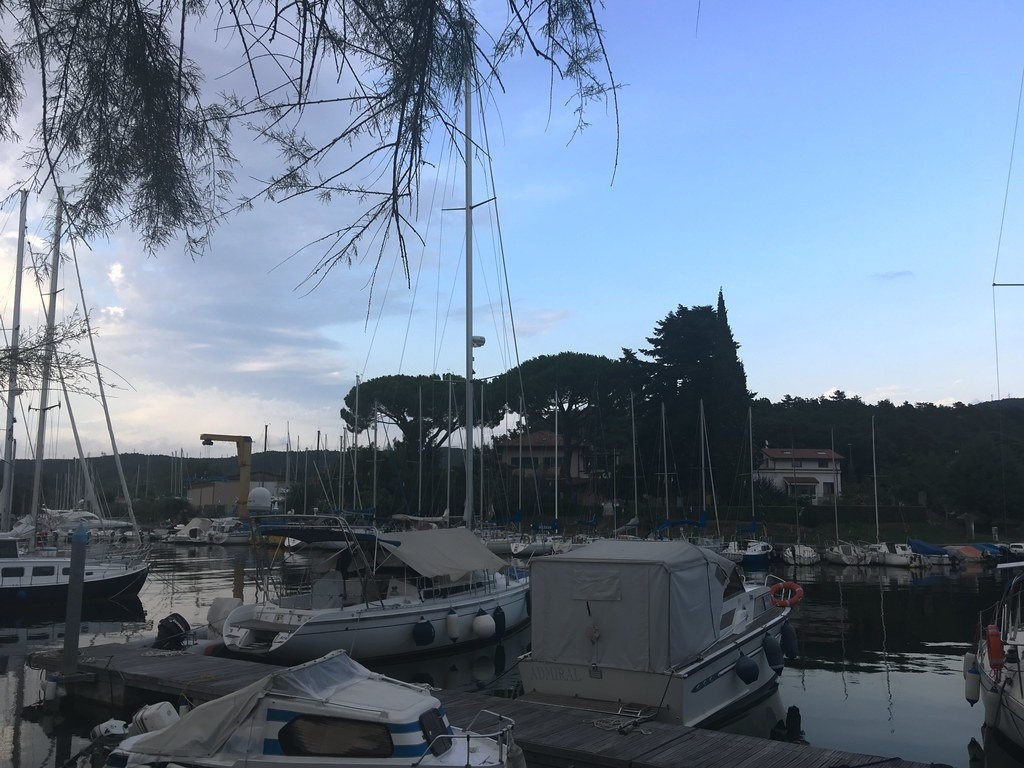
[
  {"xmin": 248, "ymin": 373, "xmax": 727, "ymax": 553},
  {"xmin": 221, "ymin": 15, "xmax": 535, "ymax": 665},
  {"xmin": 0, "ymin": 186, "xmax": 150, "ymax": 618},
  {"xmin": 782, "ymin": 443, "xmax": 820, "ymax": 566},
  {"xmin": 822, "ymin": 414, "xmax": 917, "ymax": 566},
  {"xmin": 721, "ymin": 406, "xmax": 774, "ymax": 566}
]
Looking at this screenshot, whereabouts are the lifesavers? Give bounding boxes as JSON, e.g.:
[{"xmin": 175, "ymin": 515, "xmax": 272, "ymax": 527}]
[
  {"xmin": 770, "ymin": 581, "xmax": 804, "ymax": 607},
  {"xmin": 986, "ymin": 624, "xmax": 1003, "ymax": 670}
]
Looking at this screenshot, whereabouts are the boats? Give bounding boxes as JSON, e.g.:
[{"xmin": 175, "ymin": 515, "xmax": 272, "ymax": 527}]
[
  {"xmin": 519, "ymin": 537, "xmax": 795, "ymax": 725},
  {"xmin": 206, "ymin": 519, "xmax": 251, "ymax": 544},
  {"xmin": 968, "ymin": 722, "xmax": 1024, "ymax": 768},
  {"xmin": 122, "ymin": 611, "xmax": 224, "ymax": 657},
  {"xmin": 71, "ymin": 650, "xmax": 524, "ymax": 767},
  {"xmin": 962, "ymin": 560, "xmax": 1024, "ymax": 750},
  {"xmin": 36, "ymin": 527, "xmax": 207, "ymax": 544},
  {"xmin": 910, "ymin": 537, "xmax": 1024, "ymax": 567}
]
